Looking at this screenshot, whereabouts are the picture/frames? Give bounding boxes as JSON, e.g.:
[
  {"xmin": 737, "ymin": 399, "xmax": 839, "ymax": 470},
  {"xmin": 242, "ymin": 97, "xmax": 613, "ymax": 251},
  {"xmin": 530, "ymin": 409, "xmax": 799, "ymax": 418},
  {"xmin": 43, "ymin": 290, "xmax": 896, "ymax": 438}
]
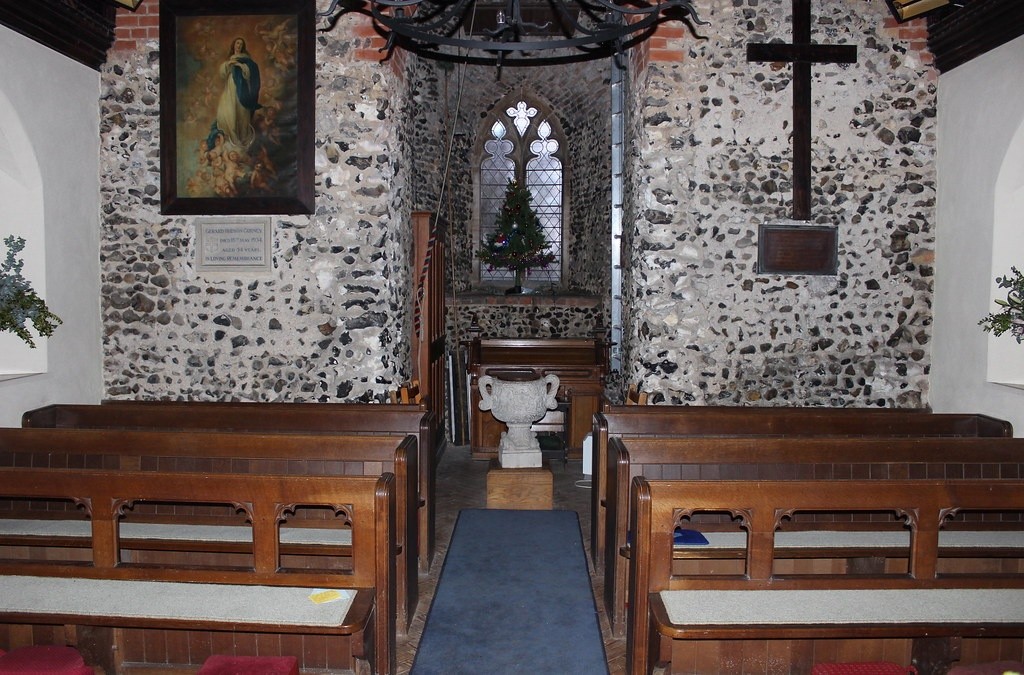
[
  {"xmin": 159, "ymin": 0, "xmax": 317, "ymax": 215},
  {"xmin": 195, "ymin": 218, "xmax": 272, "ymax": 274},
  {"xmin": 758, "ymin": 224, "xmax": 838, "ymax": 277}
]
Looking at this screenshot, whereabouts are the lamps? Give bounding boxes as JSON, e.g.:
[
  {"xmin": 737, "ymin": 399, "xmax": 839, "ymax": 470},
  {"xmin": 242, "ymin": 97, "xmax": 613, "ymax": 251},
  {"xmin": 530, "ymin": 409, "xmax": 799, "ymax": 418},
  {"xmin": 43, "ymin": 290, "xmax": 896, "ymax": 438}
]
[{"xmin": 316, "ymin": 0, "xmax": 712, "ymax": 81}]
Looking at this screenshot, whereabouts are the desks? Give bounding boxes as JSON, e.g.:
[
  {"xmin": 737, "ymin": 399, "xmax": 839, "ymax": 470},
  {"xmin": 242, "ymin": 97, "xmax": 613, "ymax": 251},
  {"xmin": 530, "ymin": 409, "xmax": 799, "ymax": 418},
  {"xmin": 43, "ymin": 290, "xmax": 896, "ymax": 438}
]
[{"xmin": 466, "ymin": 337, "xmax": 602, "ymax": 459}]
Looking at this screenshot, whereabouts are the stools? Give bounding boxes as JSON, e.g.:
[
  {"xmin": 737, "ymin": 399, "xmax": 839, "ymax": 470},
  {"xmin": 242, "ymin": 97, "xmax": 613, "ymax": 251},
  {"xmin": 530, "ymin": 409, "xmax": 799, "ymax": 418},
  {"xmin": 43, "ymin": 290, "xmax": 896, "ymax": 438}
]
[
  {"xmin": 197, "ymin": 656, "xmax": 299, "ymax": 675},
  {"xmin": 0, "ymin": 645, "xmax": 95, "ymax": 675}
]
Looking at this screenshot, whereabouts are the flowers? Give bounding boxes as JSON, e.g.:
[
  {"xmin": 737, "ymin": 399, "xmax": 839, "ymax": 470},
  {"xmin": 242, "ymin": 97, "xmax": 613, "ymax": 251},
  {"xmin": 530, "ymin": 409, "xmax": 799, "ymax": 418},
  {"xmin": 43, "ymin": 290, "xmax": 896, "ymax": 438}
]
[{"xmin": 978, "ymin": 264, "xmax": 1024, "ymax": 344}]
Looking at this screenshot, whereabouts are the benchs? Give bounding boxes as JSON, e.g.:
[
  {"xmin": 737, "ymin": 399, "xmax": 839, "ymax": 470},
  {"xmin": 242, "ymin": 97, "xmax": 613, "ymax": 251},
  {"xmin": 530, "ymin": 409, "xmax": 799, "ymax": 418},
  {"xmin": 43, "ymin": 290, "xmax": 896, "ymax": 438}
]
[
  {"xmin": 590, "ymin": 404, "xmax": 1024, "ymax": 675},
  {"xmin": 0, "ymin": 403, "xmax": 431, "ymax": 675}
]
